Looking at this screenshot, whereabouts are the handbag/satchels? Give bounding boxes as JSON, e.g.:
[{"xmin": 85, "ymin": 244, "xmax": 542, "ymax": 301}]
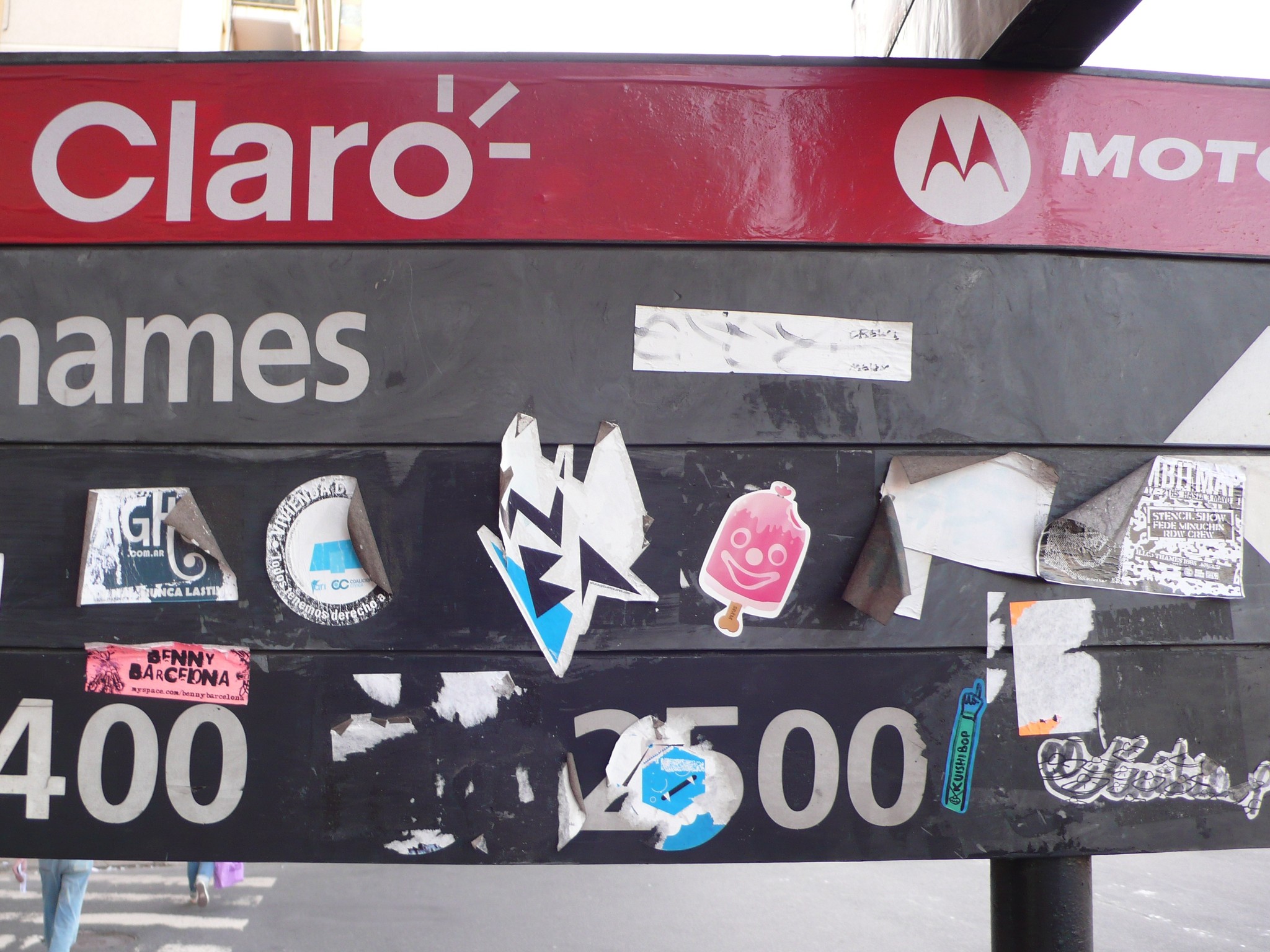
[{"xmin": 213, "ymin": 862, "xmax": 244, "ymax": 889}]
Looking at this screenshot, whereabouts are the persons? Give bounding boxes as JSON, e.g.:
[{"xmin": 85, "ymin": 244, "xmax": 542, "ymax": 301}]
[
  {"xmin": 184, "ymin": 861, "xmax": 214, "ymax": 908},
  {"xmin": 11, "ymin": 858, "xmax": 27, "ymax": 893},
  {"xmin": 38, "ymin": 858, "xmax": 94, "ymax": 952}
]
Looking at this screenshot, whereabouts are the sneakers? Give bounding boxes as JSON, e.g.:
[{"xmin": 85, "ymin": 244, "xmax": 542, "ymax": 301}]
[
  {"xmin": 189, "ymin": 888, "xmax": 199, "ymax": 903},
  {"xmin": 197, "ymin": 881, "xmax": 209, "ymax": 907}
]
